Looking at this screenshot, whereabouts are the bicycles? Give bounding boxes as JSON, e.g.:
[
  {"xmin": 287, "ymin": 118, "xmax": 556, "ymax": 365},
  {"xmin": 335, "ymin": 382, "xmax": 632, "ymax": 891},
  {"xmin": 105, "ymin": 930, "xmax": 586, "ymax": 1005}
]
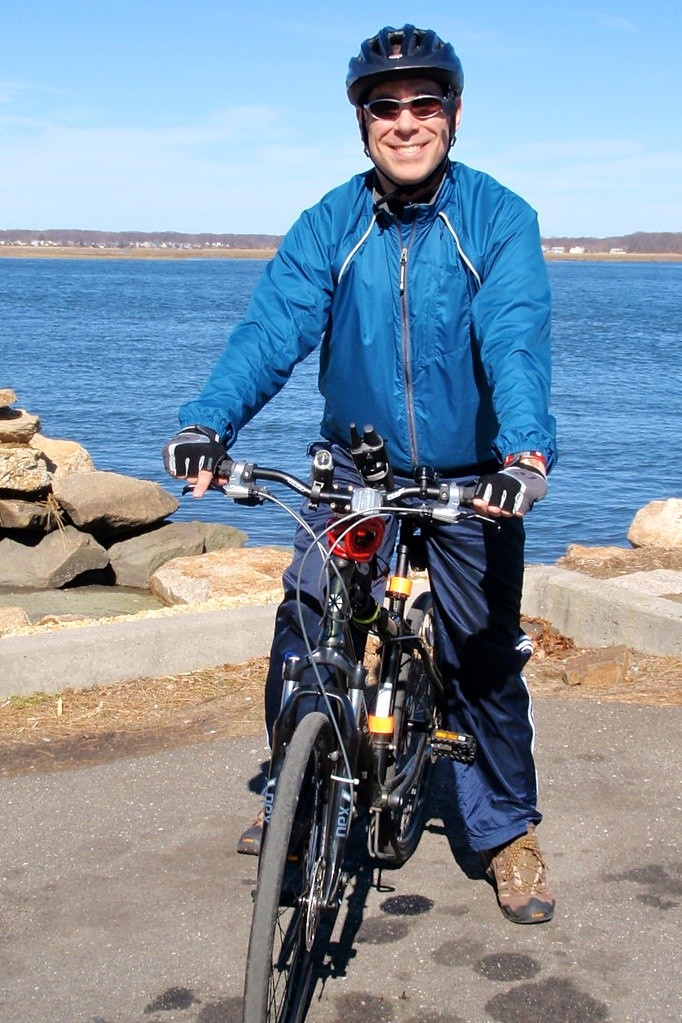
[{"xmin": 164, "ymin": 416, "xmax": 537, "ymax": 1023}]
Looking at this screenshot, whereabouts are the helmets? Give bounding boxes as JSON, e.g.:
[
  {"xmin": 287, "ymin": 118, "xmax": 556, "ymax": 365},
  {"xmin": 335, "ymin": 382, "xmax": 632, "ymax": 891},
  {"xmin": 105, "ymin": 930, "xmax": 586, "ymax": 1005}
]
[{"xmin": 345, "ymin": 24, "xmax": 464, "ymax": 107}]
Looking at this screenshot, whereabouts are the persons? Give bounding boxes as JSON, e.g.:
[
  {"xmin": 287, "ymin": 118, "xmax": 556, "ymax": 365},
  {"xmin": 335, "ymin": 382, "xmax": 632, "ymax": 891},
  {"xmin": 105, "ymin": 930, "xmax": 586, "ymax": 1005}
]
[{"xmin": 161, "ymin": 25, "xmax": 557, "ymax": 925}]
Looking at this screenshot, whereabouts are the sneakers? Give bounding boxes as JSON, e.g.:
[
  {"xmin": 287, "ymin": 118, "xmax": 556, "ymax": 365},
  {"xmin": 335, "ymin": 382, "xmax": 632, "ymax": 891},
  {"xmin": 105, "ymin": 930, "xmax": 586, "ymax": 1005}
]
[
  {"xmin": 238, "ymin": 807, "xmax": 312, "ymax": 861},
  {"xmin": 479, "ymin": 822, "xmax": 555, "ymax": 924}
]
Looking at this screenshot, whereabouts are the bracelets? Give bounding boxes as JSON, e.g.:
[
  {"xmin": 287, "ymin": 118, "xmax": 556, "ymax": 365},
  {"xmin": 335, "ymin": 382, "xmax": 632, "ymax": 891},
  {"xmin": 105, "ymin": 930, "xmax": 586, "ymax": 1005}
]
[{"xmin": 504, "ymin": 451, "xmax": 547, "ymax": 468}]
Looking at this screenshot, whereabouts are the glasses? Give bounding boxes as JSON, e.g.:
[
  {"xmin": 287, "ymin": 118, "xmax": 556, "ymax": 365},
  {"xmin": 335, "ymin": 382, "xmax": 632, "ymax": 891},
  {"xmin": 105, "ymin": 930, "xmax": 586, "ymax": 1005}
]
[{"xmin": 361, "ymin": 93, "xmax": 453, "ymax": 122}]
